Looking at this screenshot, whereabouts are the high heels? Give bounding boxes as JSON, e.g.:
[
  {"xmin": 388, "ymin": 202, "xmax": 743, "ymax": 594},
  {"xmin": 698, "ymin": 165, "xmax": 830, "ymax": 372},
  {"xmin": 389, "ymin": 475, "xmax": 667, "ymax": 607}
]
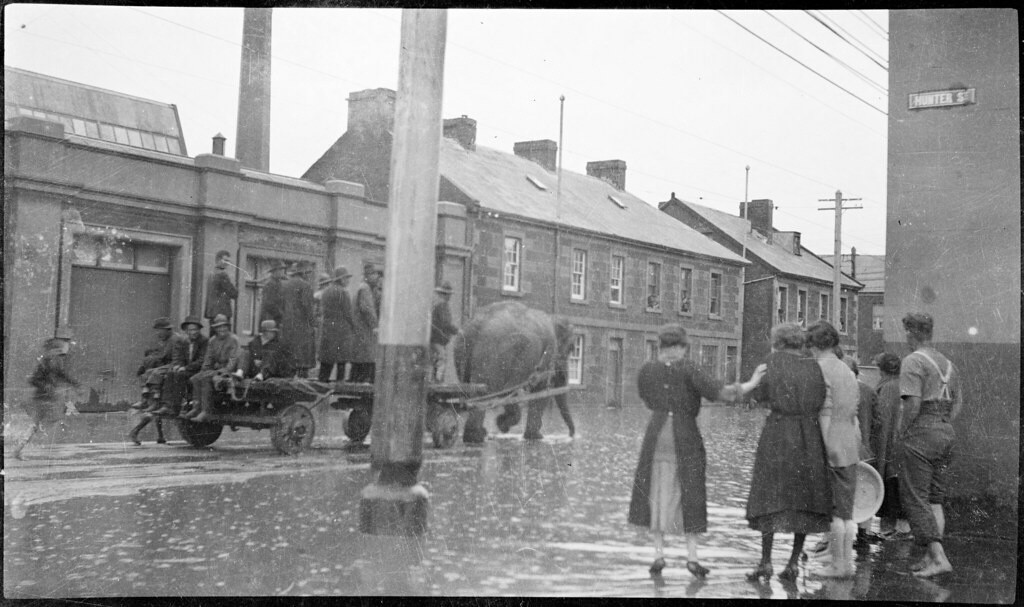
[
  {"xmin": 777, "ymin": 567, "xmax": 799, "ymax": 583},
  {"xmin": 745, "ymin": 564, "xmax": 773, "ymax": 581}
]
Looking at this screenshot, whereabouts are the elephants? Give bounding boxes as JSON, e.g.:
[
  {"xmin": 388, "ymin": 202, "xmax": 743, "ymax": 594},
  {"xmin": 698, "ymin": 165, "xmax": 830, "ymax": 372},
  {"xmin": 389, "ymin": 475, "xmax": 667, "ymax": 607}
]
[{"xmin": 454, "ymin": 296, "xmax": 577, "ymax": 443}]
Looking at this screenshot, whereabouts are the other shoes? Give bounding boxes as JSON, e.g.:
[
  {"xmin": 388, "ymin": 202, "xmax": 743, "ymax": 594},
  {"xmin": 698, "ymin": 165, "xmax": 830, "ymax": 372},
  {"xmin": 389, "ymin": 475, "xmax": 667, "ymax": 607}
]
[
  {"xmin": 190, "ymin": 410, "xmax": 215, "ymax": 422},
  {"xmin": 143, "ymin": 403, "xmax": 159, "ymax": 412},
  {"xmin": 649, "ymin": 557, "xmax": 665, "ymax": 574},
  {"xmin": 885, "ymin": 529, "xmax": 915, "ymax": 540},
  {"xmin": 153, "ymin": 406, "xmax": 176, "ymax": 415},
  {"xmin": 688, "ymin": 562, "xmax": 709, "ymax": 578},
  {"xmin": 132, "ymin": 400, "xmax": 149, "ymax": 407},
  {"xmin": 180, "ymin": 409, "xmax": 199, "ymax": 419}
]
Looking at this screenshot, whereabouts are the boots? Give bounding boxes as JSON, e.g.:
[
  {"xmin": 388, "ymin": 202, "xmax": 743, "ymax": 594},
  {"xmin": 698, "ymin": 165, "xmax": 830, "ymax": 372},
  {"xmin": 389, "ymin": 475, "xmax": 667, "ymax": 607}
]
[{"xmin": 853, "ymin": 527, "xmax": 870, "ymax": 550}]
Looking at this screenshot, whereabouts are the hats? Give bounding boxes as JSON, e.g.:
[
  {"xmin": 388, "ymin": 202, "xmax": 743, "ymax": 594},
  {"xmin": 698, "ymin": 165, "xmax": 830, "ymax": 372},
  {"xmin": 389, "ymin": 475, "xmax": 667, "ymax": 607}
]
[
  {"xmin": 875, "ymin": 351, "xmax": 901, "ymax": 373},
  {"xmin": 434, "ymin": 280, "xmax": 454, "ymax": 294},
  {"xmin": 261, "ymin": 320, "xmax": 279, "ymax": 332},
  {"xmin": 907, "ymin": 310, "xmax": 934, "ymax": 333},
  {"xmin": 364, "ymin": 265, "xmax": 383, "ymax": 279},
  {"xmin": 318, "ymin": 274, "xmax": 332, "ymax": 285},
  {"xmin": 333, "ymin": 267, "xmax": 352, "ymax": 282},
  {"xmin": 181, "ymin": 315, "xmax": 203, "ymax": 329},
  {"xmin": 46, "ymin": 328, "xmax": 71, "ymax": 340},
  {"xmin": 211, "ymin": 314, "xmax": 230, "ymax": 327},
  {"xmin": 267, "ymin": 257, "xmax": 287, "ymax": 273},
  {"xmin": 286, "ymin": 260, "xmax": 312, "ymax": 277},
  {"xmin": 153, "ymin": 318, "xmax": 174, "ymax": 328},
  {"xmin": 659, "ymin": 323, "xmax": 694, "ymax": 345}
]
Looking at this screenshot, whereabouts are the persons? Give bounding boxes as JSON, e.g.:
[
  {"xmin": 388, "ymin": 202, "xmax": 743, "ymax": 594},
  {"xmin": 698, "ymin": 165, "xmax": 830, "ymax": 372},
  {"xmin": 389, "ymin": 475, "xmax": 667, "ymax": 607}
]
[
  {"xmin": 204, "ymin": 250, "xmax": 239, "ymax": 338},
  {"xmin": 745, "ymin": 320, "xmax": 914, "ymax": 582},
  {"xmin": 259, "ymin": 254, "xmax": 384, "ymax": 384},
  {"xmin": 10, "ymin": 341, "xmax": 85, "ymax": 462},
  {"xmin": 127, "ymin": 313, "xmax": 291, "ymax": 446},
  {"xmin": 892, "ymin": 310, "xmax": 964, "ymax": 577},
  {"xmin": 431, "ymin": 278, "xmax": 460, "ymax": 387},
  {"xmin": 629, "ymin": 322, "xmax": 768, "ymax": 573}
]
